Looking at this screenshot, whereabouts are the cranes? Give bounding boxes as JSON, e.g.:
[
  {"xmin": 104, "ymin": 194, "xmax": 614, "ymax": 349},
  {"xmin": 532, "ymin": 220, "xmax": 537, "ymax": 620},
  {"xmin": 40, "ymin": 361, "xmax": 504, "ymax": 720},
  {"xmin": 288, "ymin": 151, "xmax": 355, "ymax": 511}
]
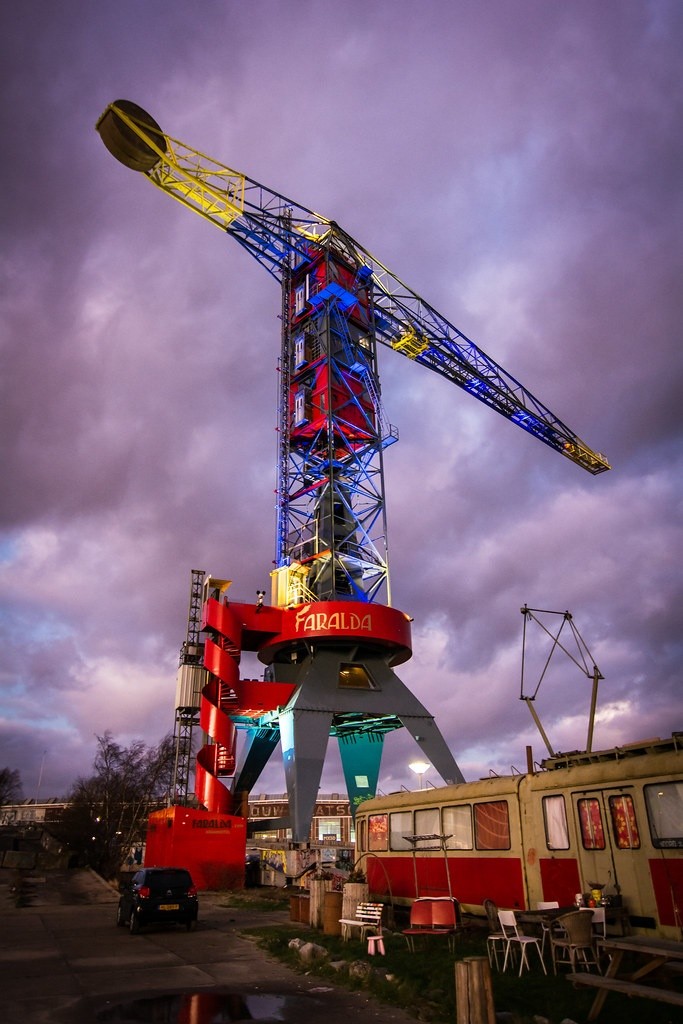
[{"xmin": 97, "ymin": 98, "xmax": 613, "ymax": 847}]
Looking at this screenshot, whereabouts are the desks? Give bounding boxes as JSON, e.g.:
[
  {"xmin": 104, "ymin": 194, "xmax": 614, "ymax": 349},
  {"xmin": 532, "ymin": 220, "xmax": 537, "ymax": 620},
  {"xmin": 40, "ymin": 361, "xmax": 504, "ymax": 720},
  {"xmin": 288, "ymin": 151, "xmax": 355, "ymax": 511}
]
[
  {"xmin": 496, "ymin": 910, "xmax": 578, "ymax": 957},
  {"xmin": 587, "ymin": 935, "xmax": 683, "ymax": 1022},
  {"xmin": 605, "ymin": 906, "xmax": 632, "ymax": 936}
]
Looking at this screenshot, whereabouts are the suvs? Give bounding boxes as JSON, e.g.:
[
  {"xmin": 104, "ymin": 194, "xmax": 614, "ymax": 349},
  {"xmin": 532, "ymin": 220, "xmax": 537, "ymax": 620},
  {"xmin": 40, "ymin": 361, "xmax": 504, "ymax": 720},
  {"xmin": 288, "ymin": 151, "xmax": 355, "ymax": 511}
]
[{"xmin": 116, "ymin": 867, "xmax": 199, "ymax": 934}]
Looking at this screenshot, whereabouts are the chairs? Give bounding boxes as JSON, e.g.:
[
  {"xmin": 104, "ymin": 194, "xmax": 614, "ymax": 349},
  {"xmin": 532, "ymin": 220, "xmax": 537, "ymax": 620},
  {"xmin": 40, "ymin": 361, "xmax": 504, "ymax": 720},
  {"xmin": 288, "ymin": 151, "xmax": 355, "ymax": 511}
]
[
  {"xmin": 484, "ymin": 899, "xmax": 520, "ymax": 965},
  {"xmin": 562, "ymin": 907, "xmax": 606, "ymax": 968},
  {"xmin": 537, "ymin": 902, "xmax": 567, "ymax": 955},
  {"xmin": 549, "ymin": 910, "xmax": 603, "ymax": 976},
  {"xmin": 497, "ymin": 910, "xmax": 547, "ymax": 977}
]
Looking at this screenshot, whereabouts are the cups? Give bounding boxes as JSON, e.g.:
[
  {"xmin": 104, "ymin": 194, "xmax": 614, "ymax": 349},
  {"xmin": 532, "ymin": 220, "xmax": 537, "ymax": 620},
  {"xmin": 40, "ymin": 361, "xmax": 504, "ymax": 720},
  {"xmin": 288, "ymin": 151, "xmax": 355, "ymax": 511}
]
[{"xmin": 589, "ymin": 900, "xmax": 596, "ymax": 908}]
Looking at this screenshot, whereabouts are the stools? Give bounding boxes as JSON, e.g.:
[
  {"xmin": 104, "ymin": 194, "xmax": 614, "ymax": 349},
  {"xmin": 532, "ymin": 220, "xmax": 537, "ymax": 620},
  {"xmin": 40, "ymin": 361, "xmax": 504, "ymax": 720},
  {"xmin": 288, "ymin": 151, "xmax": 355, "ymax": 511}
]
[
  {"xmin": 486, "ymin": 935, "xmax": 515, "ymax": 972},
  {"xmin": 367, "ymin": 936, "xmax": 385, "ymax": 956}
]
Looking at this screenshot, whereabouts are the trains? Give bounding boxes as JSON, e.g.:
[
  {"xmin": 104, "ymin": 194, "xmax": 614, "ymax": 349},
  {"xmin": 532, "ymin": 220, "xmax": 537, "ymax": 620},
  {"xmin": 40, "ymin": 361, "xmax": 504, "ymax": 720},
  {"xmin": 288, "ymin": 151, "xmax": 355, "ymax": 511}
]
[{"xmin": 350, "ymin": 729, "xmax": 683, "ymax": 944}]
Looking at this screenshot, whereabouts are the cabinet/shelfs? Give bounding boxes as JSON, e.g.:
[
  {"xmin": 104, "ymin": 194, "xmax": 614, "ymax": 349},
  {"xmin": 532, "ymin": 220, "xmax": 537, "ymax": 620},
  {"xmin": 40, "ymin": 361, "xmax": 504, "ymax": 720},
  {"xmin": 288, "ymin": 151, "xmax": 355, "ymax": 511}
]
[{"xmin": 290, "ymin": 894, "xmax": 311, "ymax": 924}]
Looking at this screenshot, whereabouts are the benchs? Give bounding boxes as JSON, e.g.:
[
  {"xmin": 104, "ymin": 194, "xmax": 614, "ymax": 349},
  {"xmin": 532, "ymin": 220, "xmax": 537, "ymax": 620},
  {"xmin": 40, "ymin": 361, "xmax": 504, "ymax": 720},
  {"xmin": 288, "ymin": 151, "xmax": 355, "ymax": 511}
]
[
  {"xmin": 339, "ymin": 902, "xmax": 383, "ymax": 944},
  {"xmin": 403, "ymin": 900, "xmax": 472, "ymax": 956},
  {"xmin": 638, "ymin": 958, "xmax": 683, "ymax": 976},
  {"xmin": 565, "ymin": 972, "xmax": 683, "ymax": 1009}
]
